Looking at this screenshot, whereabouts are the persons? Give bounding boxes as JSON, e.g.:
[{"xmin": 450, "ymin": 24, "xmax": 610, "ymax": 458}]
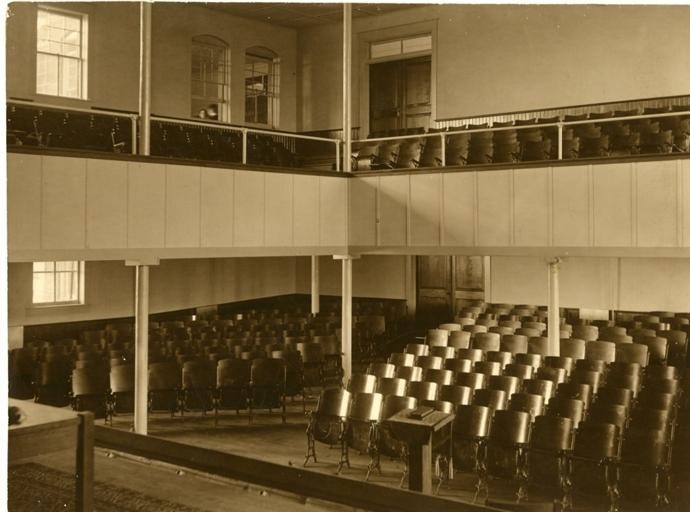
[
  {"xmin": 206, "ymin": 104, "xmax": 218, "ymax": 119},
  {"xmin": 199, "ymin": 109, "xmax": 207, "ymax": 120}
]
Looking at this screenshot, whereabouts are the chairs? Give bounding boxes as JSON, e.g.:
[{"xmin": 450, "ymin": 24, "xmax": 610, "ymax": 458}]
[
  {"xmin": 7, "ymin": 94, "xmax": 690, "ymax": 172},
  {"xmin": 8, "ymin": 297, "xmax": 690, "ymax": 511}
]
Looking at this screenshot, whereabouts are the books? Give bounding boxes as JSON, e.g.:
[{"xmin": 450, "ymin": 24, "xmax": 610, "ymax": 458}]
[
  {"xmin": 408, "ymin": 409, "xmax": 436, "ymax": 421},
  {"xmin": 411, "ymin": 406, "xmax": 434, "ymax": 418}
]
